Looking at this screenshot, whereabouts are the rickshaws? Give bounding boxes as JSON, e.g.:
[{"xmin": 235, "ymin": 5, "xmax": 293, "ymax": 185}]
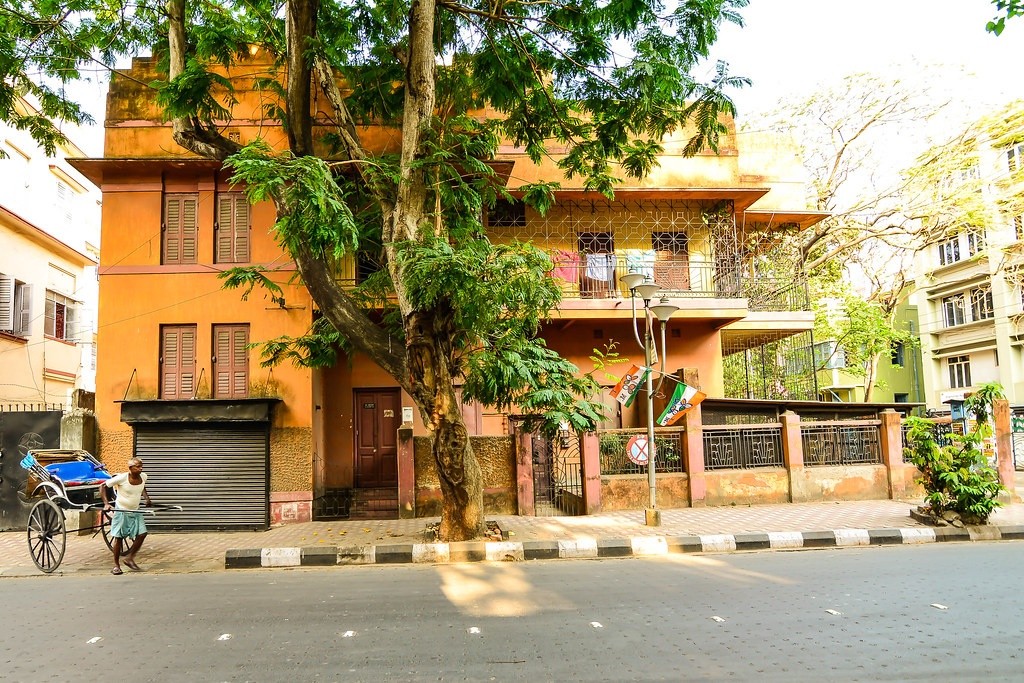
[{"xmin": 17, "ymin": 448, "xmax": 183, "ymax": 573}]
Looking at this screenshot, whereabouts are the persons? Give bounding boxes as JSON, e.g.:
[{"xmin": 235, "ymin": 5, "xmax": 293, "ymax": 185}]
[{"xmin": 99, "ymin": 457, "xmax": 153, "ymax": 575}]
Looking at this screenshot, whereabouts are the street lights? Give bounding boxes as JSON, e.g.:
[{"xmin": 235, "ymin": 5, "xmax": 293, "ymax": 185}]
[{"xmin": 619, "ymin": 265, "xmax": 680, "ymax": 527}]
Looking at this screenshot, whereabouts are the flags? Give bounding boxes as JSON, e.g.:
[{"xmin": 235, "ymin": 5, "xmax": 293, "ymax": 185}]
[
  {"xmin": 608, "ymin": 364, "xmax": 653, "ymax": 408},
  {"xmin": 655, "ymin": 380, "xmax": 708, "ymax": 427}
]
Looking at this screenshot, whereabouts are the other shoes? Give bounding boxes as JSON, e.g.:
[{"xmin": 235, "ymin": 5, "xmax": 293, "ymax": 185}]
[
  {"xmin": 124, "ymin": 559, "xmax": 139, "ymax": 570},
  {"xmin": 110, "ymin": 566, "xmax": 123, "ymax": 575}
]
